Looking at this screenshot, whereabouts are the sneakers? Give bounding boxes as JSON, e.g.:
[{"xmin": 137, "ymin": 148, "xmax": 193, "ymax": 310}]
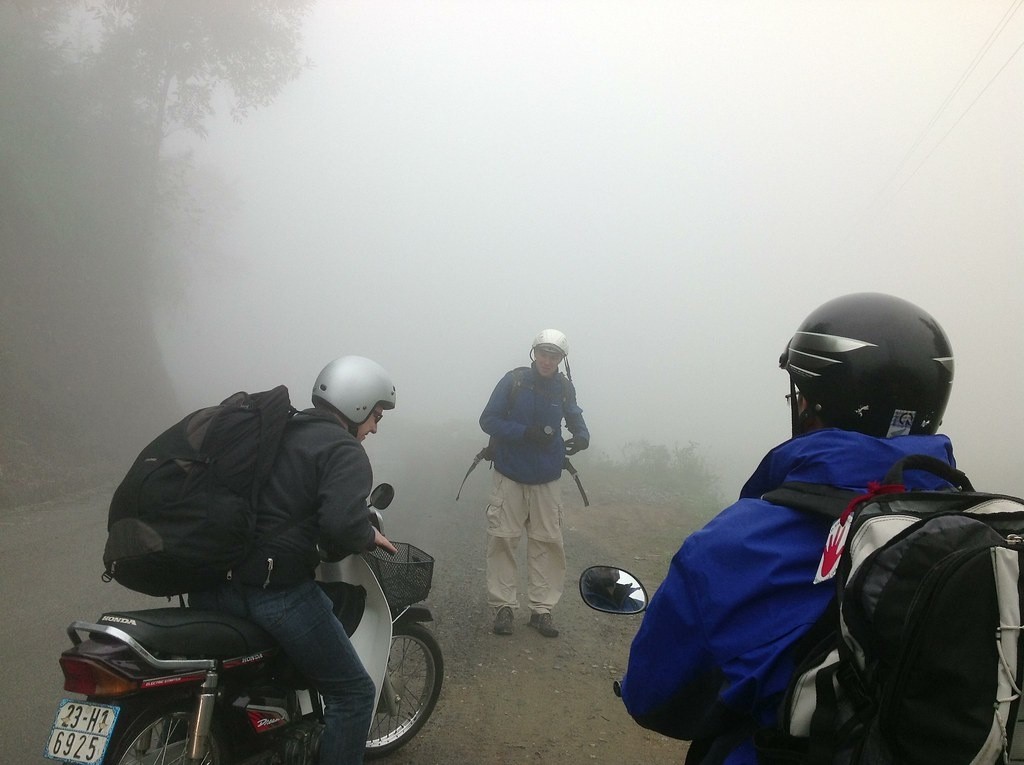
[
  {"xmin": 531, "ymin": 613, "xmax": 558, "ymax": 637},
  {"xmin": 495, "ymin": 607, "xmax": 514, "ymax": 634}
]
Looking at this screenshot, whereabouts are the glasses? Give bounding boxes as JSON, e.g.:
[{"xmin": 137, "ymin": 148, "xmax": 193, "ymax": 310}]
[
  {"xmin": 785, "ymin": 391, "xmax": 804, "ymax": 415},
  {"xmin": 372, "ymin": 409, "xmax": 383, "ymax": 424}
]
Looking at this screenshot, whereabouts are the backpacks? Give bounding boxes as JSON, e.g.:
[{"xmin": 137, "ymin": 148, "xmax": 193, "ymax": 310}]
[
  {"xmin": 101, "ymin": 384, "xmax": 346, "ymax": 588},
  {"xmin": 763, "ymin": 454, "xmax": 1024, "ymax": 765}
]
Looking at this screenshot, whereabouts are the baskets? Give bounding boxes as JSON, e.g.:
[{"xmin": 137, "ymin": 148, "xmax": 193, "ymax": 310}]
[{"xmin": 360, "ymin": 541, "xmax": 436, "ymax": 608}]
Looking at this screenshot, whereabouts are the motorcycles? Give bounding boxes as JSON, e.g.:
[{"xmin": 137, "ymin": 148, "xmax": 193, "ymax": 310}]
[
  {"xmin": 43, "ymin": 483, "xmax": 444, "ymax": 765},
  {"xmin": 578, "ymin": 559, "xmax": 657, "ymax": 698}
]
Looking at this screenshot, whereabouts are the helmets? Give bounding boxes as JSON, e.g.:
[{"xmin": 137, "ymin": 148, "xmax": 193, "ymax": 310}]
[
  {"xmin": 312, "ymin": 355, "xmax": 396, "ymax": 423},
  {"xmin": 533, "ymin": 329, "xmax": 568, "ymax": 355},
  {"xmin": 778, "ymin": 292, "xmax": 955, "ymax": 439}
]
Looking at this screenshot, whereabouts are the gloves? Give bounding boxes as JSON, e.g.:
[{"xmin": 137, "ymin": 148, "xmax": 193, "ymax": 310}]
[
  {"xmin": 524, "ymin": 427, "xmax": 555, "ymax": 441},
  {"xmin": 563, "ymin": 436, "xmax": 589, "ymax": 455}
]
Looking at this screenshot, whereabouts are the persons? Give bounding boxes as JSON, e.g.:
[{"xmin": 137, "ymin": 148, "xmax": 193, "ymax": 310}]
[
  {"xmin": 456, "ymin": 327, "xmax": 593, "ymax": 637},
  {"xmin": 619, "ymin": 289, "xmax": 1023, "ymax": 765},
  {"xmin": 187, "ymin": 352, "xmax": 400, "ymax": 764}
]
